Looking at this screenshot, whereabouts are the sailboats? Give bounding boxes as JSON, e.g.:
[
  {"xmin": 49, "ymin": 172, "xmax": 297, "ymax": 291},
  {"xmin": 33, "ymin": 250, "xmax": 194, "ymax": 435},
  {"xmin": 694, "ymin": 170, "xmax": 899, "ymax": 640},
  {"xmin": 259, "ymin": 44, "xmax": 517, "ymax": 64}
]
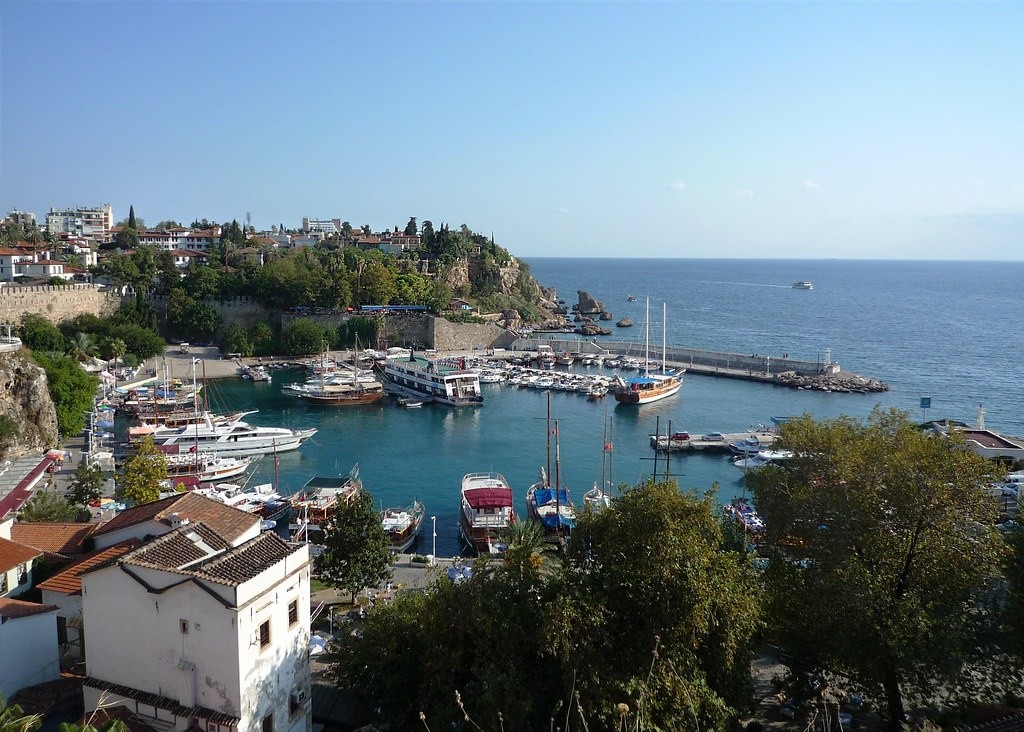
[
  {"xmin": 125, "ymin": 353, "xmax": 203, "ymax": 414},
  {"xmin": 615, "ymin": 297, "xmax": 686, "ymax": 405},
  {"xmin": 253, "ymin": 437, "xmax": 291, "ymax": 521},
  {"xmin": 583, "ymin": 402, "xmax": 613, "ymax": 515},
  {"xmin": 282, "ymin": 332, "xmax": 384, "ymax": 404},
  {"xmin": 162, "ymin": 356, "xmax": 255, "ymax": 481},
  {"xmin": 525, "ymin": 391, "xmax": 580, "ymax": 533}
]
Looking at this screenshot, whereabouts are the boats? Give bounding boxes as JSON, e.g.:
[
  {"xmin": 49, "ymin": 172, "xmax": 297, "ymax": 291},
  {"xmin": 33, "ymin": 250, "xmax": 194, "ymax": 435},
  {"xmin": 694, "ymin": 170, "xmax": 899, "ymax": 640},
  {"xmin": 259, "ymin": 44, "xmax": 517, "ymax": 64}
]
[
  {"xmin": 792, "ymin": 281, "xmax": 812, "ymax": 289},
  {"xmin": 734, "ymin": 450, "xmax": 823, "ymax": 476},
  {"xmin": 153, "ymin": 411, "xmax": 318, "ymax": 457},
  {"xmin": 638, "ymin": 361, "xmax": 661, "ymax": 371},
  {"xmin": 536, "ymin": 345, "xmax": 556, "ymax": 369},
  {"xmin": 459, "ymin": 472, "xmax": 515, "ymax": 557},
  {"xmin": 385, "ymin": 349, "xmax": 484, "ymax": 407},
  {"xmin": 404, "ymin": 400, "xmax": 423, "ymax": 406},
  {"xmin": 728, "ymin": 435, "xmax": 780, "ymax": 455},
  {"xmin": 556, "ymin": 352, "xmax": 574, "ymax": 365},
  {"xmin": 288, "ymin": 476, "xmax": 363, "ymax": 538},
  {"xmin": 593, "ymin": 355, "xmax": 604, "ymax": 365},
  {"xmin": 582, "ymin": 355, "xmax": 593, "ymax": 364},
  {"xmin": 379, "ymin": 500, "xmax": 425, "ymax": 554},
  {"xmin": 479, "ymin": 366, "xmax": 627, "ymax": 400},
  {"xmin": 605, "ymin": 358, "xmax": 640, "ymax": 370}
]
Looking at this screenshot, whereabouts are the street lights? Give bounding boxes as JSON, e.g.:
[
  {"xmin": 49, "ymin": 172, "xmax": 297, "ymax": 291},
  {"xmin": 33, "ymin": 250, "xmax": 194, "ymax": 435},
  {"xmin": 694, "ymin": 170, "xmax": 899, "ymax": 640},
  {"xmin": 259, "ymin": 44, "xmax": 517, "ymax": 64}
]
[
  {"xmin": 329, "ymin": 606, "xmax": 333, "ymax": 635},
  {"xmin": 766, "ymin": 357, "xmax": 769, "ymax": 373},
  {"xmin": 431, "ymin": 516, "xmax": 435, "ymax": 564}
]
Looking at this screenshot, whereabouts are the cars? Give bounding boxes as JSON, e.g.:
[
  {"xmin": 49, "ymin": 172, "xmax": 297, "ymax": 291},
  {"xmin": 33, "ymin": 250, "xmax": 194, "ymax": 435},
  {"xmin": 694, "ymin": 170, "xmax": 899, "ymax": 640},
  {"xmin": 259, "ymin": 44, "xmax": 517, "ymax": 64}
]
[
  {"xmin": 1002, "ymin": 483, "xmax": 1019, "ymax": 496},
  {"xmin": 671, "ymin": 431, "xmax": 690, "ymax": 440},
  {"xmin": 703, "ymin": 432, "xmax": 724, "ymax": 441},
  {"xmin": 1011, "ymin": 484, "xmax": 1024, "ymax": 498}
]
